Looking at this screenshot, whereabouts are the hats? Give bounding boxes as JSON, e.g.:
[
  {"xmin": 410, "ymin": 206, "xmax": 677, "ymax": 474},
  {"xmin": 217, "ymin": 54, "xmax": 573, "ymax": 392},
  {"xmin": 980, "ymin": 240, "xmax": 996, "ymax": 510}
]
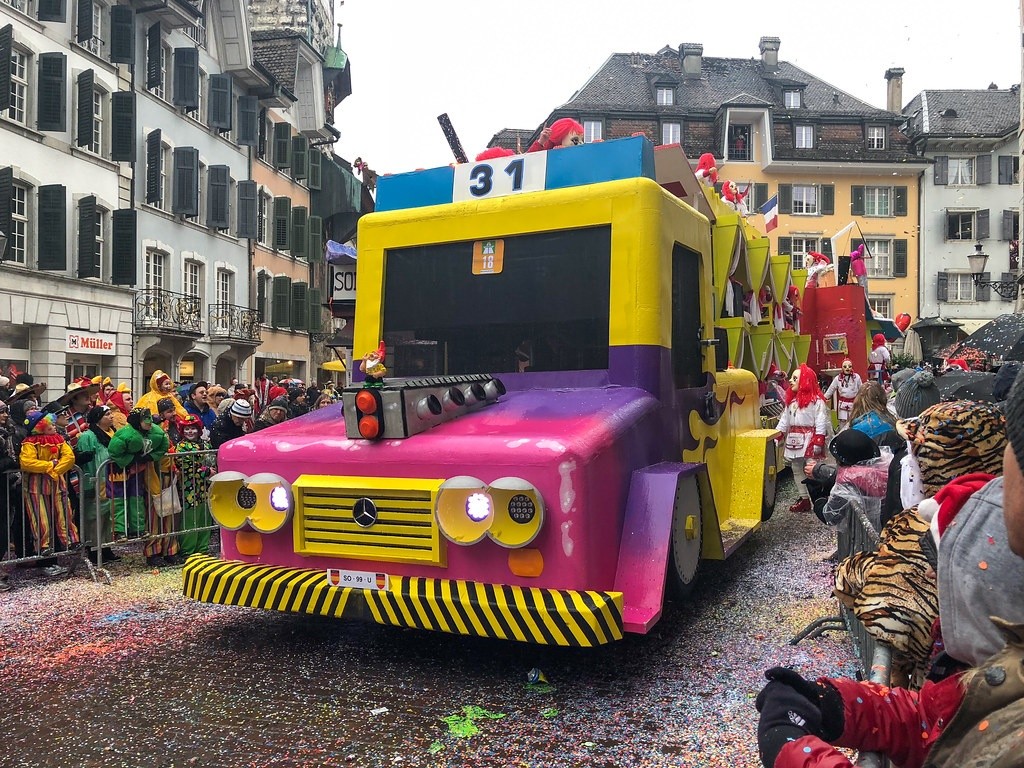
[
  {"xmin": 0, "ymin": 375, "xmax": 303, "ymax": 435},
  {"xmin": 894, "ymin": 372, "xmax": 941, "ymax": 418},
  {"xmin": 1005, "ymin": 363, "xmax": 1024, "ymax": 474},
  {"xmin": 828, "ymin": 429, "xmax": 881, "ymax": 466}
]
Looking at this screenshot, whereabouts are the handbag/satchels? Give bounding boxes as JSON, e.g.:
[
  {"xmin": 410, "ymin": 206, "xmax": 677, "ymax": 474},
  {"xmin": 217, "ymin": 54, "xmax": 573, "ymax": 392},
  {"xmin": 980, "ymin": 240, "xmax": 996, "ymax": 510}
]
[{"xmin": 152, "ymin": 484, "xmax": 182, "ymax": 518}]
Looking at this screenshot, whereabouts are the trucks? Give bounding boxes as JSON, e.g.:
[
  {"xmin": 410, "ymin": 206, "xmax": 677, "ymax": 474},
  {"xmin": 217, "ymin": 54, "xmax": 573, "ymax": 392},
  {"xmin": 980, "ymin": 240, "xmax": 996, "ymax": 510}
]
[{"xmin": 182, "ymin": 134, "xmax": 812, "ymax": 647}]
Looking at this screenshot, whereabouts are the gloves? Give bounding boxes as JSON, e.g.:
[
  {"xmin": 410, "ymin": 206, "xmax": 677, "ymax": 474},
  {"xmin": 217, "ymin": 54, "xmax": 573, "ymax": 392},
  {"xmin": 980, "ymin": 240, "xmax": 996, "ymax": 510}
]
[
  {"xmin": 801, "ymin": 477, "xmax": 823, "ymax": 502},
  {"xmin": 755, "ymin": 666, "xmax": 844, "ymax": 744},
  {"xmin": 814, "ymin": 444, "xmax": 821, "ymax": 455},
  {"xmin": 758, "ymin": 684, "xmax": 823, "ymax": 767},
  {"xmin": 773, "ymin": 438, "xmax": 779, "ymax": 447}
]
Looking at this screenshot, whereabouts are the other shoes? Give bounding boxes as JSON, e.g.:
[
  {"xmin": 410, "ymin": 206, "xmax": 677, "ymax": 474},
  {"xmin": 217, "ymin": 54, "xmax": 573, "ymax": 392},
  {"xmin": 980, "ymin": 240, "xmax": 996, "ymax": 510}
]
[
  {"xmin": 114, "ymin": 533, "xmax": 127, "ymax": 543},
  {"xmin": 0, "ymin": 541, "xmax": 84, "ymax": 592},
  {"xmin": 790, "ymin": 496, "xmax": 811, "ymax": 512},
  {"xmin": 147, "ymin": 553, "xmax": 185, "ymax": 567},
  {"xmin": 128, "ymin": 530, "xmax": 151, "ymax": 539},
  {"xmin": 87, "ymin": 545, "xmax": 123, "ymax": 565}
]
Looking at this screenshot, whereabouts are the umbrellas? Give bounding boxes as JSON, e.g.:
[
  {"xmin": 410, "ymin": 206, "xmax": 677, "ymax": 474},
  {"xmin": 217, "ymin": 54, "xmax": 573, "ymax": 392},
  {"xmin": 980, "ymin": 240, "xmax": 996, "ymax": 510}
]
[
  {"xmin": 936, "ymin": 312, "xmax": 1024, "ymax": 405},
  {"xmin": 279, "ymin": 378, "xmax": 304, "ymax": 384}
]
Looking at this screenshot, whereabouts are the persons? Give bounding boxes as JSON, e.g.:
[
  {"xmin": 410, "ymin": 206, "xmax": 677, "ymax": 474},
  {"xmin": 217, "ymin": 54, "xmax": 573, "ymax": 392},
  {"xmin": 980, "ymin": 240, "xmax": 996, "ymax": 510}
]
[
  {"xmin": 694, "ymin": 152, "xmax": 718, "ymax": 187},
  {"xmin": 335, "ymin": 381, "xmax": 344, "ymax": 396},
  {"xmin": 742, "ymin": 285, "xmax": 803, "ymax": 333},
  {"xmin": 316, "ymin": 394, "xmax": 331, "ymax": 409},
  {"xmin": 0, "ymin": 359, "xmax": 189, "ymax": 593},
  {"xmin": 109, "ymin": 407, "xmax": 169, "ymax": 543},
  {"xmin": 871, "ymin": 333, "xmax": 890, "ymax": 382},
  {"xmin": 323, "ymin": 381, "xmax": 338, "ymax": 404},
  {"xmin": 756, "ymin": 363, "xmax": 1024, "ymax": 767},
  {"xmin": 256, "ymin": 373, "xmax": 273, "ymax": 408},
  {"xmin": 526, "ymin": 118, "xmax": 583, "ymax": 151},
  {"xmin": 806, "ymin": 252, "xmax": 834, "ymax": 289},
  {"xmin": 176, "ymin": 377, "xmax": 256, "ymax": 452},
  {"xmin": 774, "ymin": 363, "xmax": 827, "ymax": 513},
  {"xmin": 720, "ymin": 180, "xmax": 752, "ymax": 216},
  {"xmin": 287, "ymin": 387, "xmax": 310, "ymax": 420},
  {"xmin": 824, "ymin": 358, "xmax": 862, "ymax": 430},
  {"xmin": 308, "ymin": 381, "xmax": 321, "ymax": 407},
  {"xmin": 173, "ymin": 414, "xmax": 217, "ymax": 565},
  {"xmin": 78, "ymin": 405, "xmax": 122, "ymax": 564},
  {"xmin": 254, "ymin": 396, "xmax": 288, "ymax": 432}
]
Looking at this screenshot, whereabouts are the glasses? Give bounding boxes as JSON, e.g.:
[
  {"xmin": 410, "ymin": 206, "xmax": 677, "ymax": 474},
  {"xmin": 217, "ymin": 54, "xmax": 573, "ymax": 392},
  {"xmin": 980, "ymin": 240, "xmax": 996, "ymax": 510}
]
[
  {"xmin": 56, "ymin": 410, "xmax": 70, "ymax": 417},
  {"xmin": 199, "ymin": 390, "xmax": 209, "ymax": 395},
  {"xmin": 0, "ymin": 409, "xmax": 8, "ymax": 414}
]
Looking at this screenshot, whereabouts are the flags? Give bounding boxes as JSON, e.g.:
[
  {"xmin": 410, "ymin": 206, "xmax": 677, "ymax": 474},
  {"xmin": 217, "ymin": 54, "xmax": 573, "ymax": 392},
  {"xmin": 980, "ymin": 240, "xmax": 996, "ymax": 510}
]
[{"xmin": 761, "ymin": 195, "xmax": 778, "ymax": 233}]
